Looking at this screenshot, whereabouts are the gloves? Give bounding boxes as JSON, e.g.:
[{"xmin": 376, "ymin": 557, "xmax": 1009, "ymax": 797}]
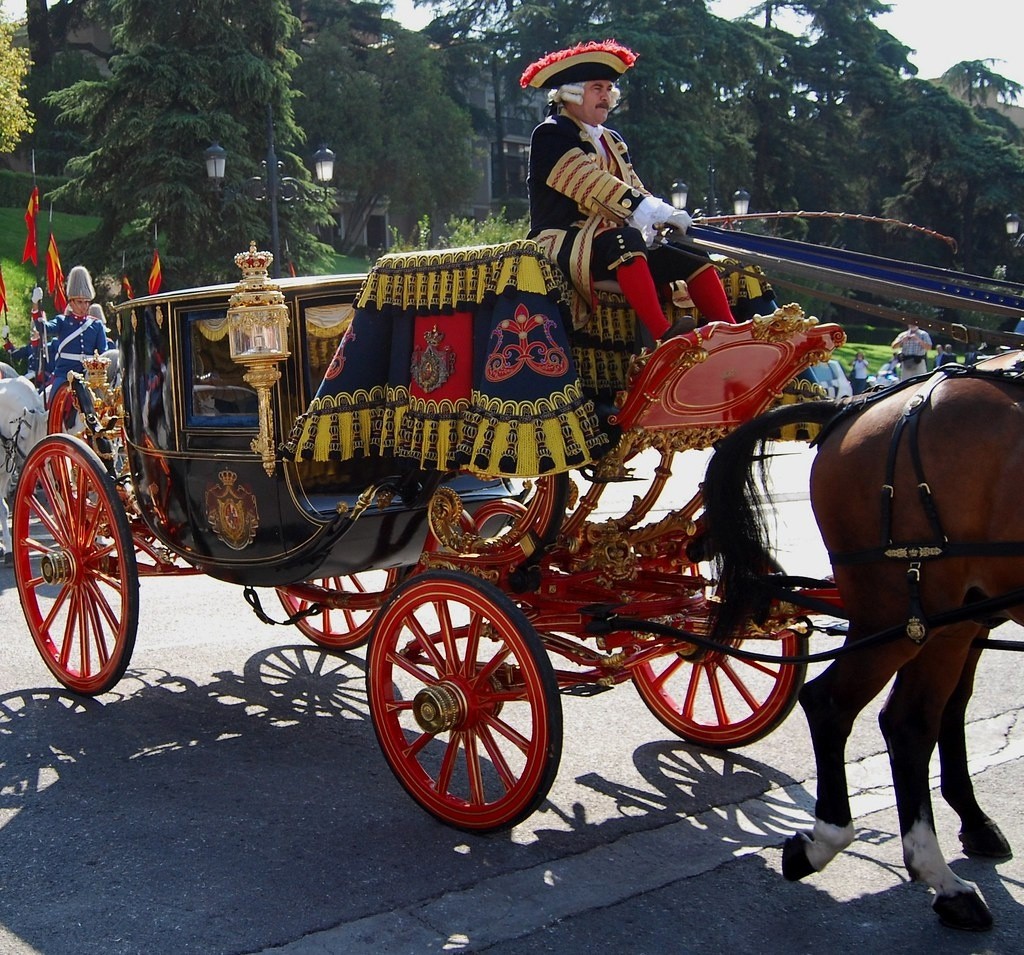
[
  {"xmin": 668, "ymin": 209, "xmax": 693, "ymax": 236},
  {"xmin": 32, "ymin": 285, "xmax": 44, "ymax": 304},
  {"xmin": 4, "ymin": 326, "xmax": 10, "ymax": 339}
]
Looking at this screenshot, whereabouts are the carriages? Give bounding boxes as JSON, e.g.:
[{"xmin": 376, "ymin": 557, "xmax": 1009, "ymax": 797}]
[{"xmin": 0, "ymin": 240, "xmax": 1023, "ymax": 935}]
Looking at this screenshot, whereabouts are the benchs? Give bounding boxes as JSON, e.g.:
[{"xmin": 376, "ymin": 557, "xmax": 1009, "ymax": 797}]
[
  {"xmin": 194, "ymin": 385, "xmax": 259, "ymax": 433},
  {"xmin": 501, "ymin": 238, "xmax": 770, "ymax": 394}
]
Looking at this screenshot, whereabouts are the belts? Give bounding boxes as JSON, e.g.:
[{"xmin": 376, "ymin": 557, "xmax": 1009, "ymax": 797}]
[{"xmin": 60, "ymin": 352, "xmax": 95, "ymax": 363}]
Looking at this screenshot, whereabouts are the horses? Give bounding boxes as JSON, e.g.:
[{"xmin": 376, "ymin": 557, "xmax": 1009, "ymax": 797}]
[
  {"xmin": 0, "ymin": 350, "xmax": 122, "ymax": 566},
  {"xmin": 673, "ymin": 365, "xmax": 1024, "ymax": 927}
]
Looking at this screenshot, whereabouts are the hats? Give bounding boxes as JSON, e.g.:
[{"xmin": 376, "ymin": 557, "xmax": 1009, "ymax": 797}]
[
  {"xmin": 67, "ymin": 267, "xmax": 95, "ymax": 303},
  {"xmin": 519, "ymin": 39, "xmax": 638, "ymax": 87},
  {"xmin": 89, "ymin": 303, "xmax": 108, "ymax": 327},
  {"xmin": 30, "ymin": 317, "xmax": 36, "ymax": 332}
]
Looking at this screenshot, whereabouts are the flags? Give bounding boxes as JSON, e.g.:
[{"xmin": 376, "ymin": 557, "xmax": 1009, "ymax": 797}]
[
  {"xmin": 22, "ymin": 186, "xmax": 39, "ymax": 264},
  {"xmin": 147, "ymin": 250, "xmax": 162, "ymax": 295},
  {"xmin": 44, "ymin": 231, "xmax": 66, "ymax": 311},
  {"xmin": 0, "ymin": 272, "xmax": 6, "ymax": 314},
  {"xmin": 123, "ymin": 274, "xmax": 135, "ymax": 300}
]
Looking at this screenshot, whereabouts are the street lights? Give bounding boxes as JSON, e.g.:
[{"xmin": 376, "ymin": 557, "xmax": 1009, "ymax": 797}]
[
  {"xmin": 204, "ymin": 99, "xmax": 336, "ymax": 279},
  {"xmin": 670, "ymin": 162, "xmax": 752, "ymax": 219}
]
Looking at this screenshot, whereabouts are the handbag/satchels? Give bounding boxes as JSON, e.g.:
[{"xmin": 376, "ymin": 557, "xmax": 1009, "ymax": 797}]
[{"xmin": 851, "ymin": 369, "xmax": 856, "ymax": 378}]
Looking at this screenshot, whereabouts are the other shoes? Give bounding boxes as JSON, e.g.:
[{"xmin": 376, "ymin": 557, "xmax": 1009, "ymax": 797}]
[{"xmin": 660, "ymin": 315, "xmax": 696, "ymax": 344}]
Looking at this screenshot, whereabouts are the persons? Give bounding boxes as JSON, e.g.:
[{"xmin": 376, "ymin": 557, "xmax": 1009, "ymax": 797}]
[
  {"xmin": 89, "ymin": 303, "xmax": 116, "ymax": 350},
  {"xmin": 0, "ymin": 312, "xmax": 55, "ymax": 381},
  {"xmin": 964, "ymin": 345, "xmax": 982, "ymax": 364},
  {"xmin": 32, "ymin": 266, "xmax": 107, "ymax": 429},
  {"xmin": 147, "ymin": 343, "xmax": 169, "ymax": 437},
  {"xmin": 850, "ymin": 352, "xmax": 870, "ymax": 380},
  {"xmin": 935, "ymin": 344, "xmax": 957, "ymax": 367},
  {"xmin": 891, "ymin": 325, "xmax": 933, "ymax": 382},
  {"xmin": 520, "ymin": 40, "xmax": 737, "ymax": 344}
]
